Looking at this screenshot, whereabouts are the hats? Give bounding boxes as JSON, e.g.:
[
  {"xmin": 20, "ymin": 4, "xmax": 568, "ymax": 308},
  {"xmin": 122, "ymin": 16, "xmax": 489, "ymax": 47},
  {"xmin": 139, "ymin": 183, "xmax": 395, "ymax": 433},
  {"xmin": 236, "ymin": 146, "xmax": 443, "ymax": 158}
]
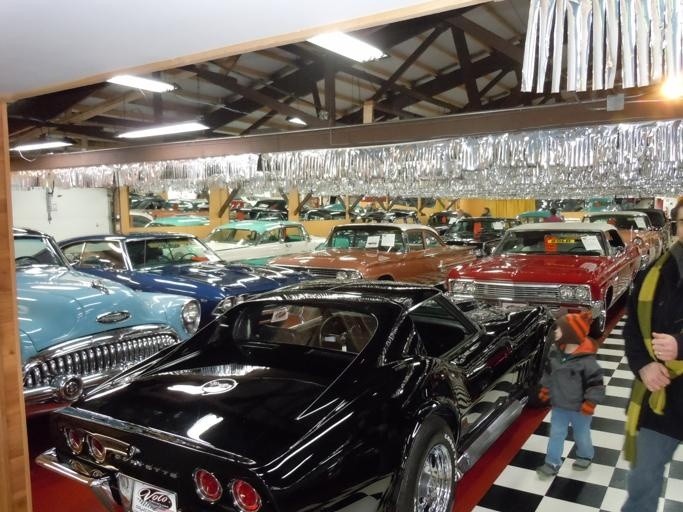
[{"xmin": 556, "ymin": 310, "xmax": 593, "ymax": 347}]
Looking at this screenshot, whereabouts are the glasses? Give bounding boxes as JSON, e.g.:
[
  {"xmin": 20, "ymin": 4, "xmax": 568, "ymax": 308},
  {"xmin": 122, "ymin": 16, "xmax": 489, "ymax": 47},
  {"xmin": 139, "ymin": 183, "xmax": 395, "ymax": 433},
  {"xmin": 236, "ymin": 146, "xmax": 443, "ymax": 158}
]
[{"xmin": 666, "ymin": 216, "xmax": 682, "ymax": 226}]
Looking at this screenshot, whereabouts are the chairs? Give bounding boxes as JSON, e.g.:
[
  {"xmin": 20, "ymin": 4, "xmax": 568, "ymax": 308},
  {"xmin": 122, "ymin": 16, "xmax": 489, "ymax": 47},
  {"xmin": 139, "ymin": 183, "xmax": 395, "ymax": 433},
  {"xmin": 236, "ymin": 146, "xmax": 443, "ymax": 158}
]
[{"xmin": 523, "ymin": 242, "xmax": 554, "ymax": 252}]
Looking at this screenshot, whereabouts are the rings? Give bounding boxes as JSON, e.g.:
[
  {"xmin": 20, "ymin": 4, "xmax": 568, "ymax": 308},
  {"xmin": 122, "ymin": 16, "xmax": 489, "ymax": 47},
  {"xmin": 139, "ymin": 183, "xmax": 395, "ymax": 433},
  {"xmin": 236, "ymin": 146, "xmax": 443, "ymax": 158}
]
[{"xmin": 658, "ymin": 351, "xmax": 661, "ymax": 357}]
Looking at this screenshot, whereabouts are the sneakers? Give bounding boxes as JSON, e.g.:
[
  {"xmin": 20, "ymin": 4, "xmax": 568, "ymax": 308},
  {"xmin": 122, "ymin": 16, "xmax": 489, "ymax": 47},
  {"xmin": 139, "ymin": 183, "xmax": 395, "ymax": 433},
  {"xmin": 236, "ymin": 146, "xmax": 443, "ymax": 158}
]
[
  {"xmin": 536, "ymin": 462, "xmax": 561, "ymax": 475},
  {"xmin": 572, "ymin": 456, "xmax": 592, "ymax": 471}
]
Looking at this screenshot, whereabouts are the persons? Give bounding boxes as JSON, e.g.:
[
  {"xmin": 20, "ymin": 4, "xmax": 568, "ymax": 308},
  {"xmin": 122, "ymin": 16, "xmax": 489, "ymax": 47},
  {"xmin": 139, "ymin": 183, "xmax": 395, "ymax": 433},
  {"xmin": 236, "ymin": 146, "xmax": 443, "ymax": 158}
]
[
  {"xmin": 621, "ymin": 196, "xmax": 683, "ymax": 511},
  {"xmin": 536, "ymin": 312, "xmax": 606, "ymax": 480},
  {"xmin": 543, "ymin": 207, "xmax": 560, "ymax": 223},
  {"xmin": 481, "ymin": 207, "xmax": 488, "ymax": 215}
]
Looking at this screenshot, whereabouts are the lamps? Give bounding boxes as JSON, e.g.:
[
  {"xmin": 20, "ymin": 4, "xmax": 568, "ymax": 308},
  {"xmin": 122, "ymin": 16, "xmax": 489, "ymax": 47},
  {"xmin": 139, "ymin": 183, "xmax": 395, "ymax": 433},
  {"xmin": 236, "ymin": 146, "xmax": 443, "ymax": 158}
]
[
  {"xmin": 112, "ymin": 70, "xmax": 212, "ymax": 139},
  {"xmin": 106, "ymin": 73, "xmax": 181, "ymax": 94},
  {"xmin": 285, "ymin": 98, "xmax": 309, "ymax": 126},
  {"xmin": 9, "ymin": 117, "xmax": 74, "ymax": 152},
  {"xmin": 305, "ymin": 28, "xmax": 390, "ymax": 64}
]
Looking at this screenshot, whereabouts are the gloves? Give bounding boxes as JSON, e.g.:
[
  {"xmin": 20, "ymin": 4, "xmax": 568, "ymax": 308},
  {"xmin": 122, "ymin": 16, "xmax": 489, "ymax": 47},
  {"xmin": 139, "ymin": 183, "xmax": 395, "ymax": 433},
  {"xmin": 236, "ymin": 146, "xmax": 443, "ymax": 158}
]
[
  {"xmin": 537, "ymin": 385, "xmax": 550, "ymax": 402},
  {"xmin": 581, "ymin": 400, "xmax": 596, "ymax": 417}
]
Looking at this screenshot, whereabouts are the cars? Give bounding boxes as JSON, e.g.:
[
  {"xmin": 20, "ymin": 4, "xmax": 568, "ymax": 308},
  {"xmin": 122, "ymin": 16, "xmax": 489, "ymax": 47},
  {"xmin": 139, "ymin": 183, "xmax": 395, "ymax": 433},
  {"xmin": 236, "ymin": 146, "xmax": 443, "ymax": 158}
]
[
  {"xmin": 31, "ymin": 230, "xmax": 311, "ymax": 330},
  {"xmin": 33, "ymin": 280, "xmax": 558, "ymax": 512},
  {"xmin": 116, "ymin": 194, "xmax": 673, "ymax": 258},
  {"xmin": 161, "ymin": 220, "xmax": 350, "ymax": 270},
  {"xmin": 12, "ymin": 227, "xmax": 202, "ymax": 443},
  {"xmin": 580, "ymin": 211, "xmax": 664, "ymax": 279},
  {"xmin": 267, "ymin": 221, "xmax": 483, "ymax": 289},
  {"xmin": 443, "ymin": 220, "xmax": 651, "ymax": 340}
]
